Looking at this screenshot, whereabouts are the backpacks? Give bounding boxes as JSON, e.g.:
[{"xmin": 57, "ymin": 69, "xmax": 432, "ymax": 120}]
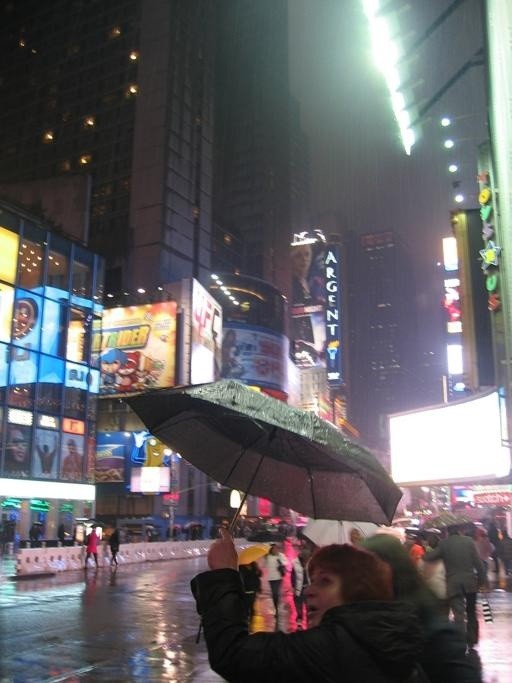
[{"xmin": 244, "ymin": 568, "xmax": 259, "ymax": 591}]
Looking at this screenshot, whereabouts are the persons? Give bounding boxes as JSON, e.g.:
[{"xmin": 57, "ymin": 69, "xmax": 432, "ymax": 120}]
[
  {"xmin": 291, "ymin": 245, "xmax": 314, "ymax": 305},
  {"xmin": 110, "ymin": 527, "xmax": 121, "ymax": 565},
  {"xmin": 219, "ymin": 331, "xmax": 237, "ymax": 378},
  {"xmin": 37, "ymin": 437, "xmax": 57, "ymax": 478},
  {"xmin": 5, "ymin": 426, "xmax": 28, "ymax": 478},
  {"xmin": 144, "ymin": 503, "xmax": 511, "ymax": 682},
  {"xmin": 63, "ymin": 440, "xmax": 86, "ymax": 481},
  {"xmin": 84, "ymin": 528, "xmax": 101, "ymax": 570}
]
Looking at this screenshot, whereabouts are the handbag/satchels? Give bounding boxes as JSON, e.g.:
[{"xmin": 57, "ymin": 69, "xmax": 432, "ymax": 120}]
[
  {"xmin": 482, "ymin": 601, "xmax": 492, "ymax": 623},
  {"xmin": 277, "ymin": 564, "xmax": 286, "ymax": 575}
]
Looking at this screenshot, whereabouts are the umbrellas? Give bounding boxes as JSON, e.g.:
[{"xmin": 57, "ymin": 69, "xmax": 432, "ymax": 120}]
[{"xmin": 121, "ymin": 380, "xmax": 404, "ymax": 532}]
